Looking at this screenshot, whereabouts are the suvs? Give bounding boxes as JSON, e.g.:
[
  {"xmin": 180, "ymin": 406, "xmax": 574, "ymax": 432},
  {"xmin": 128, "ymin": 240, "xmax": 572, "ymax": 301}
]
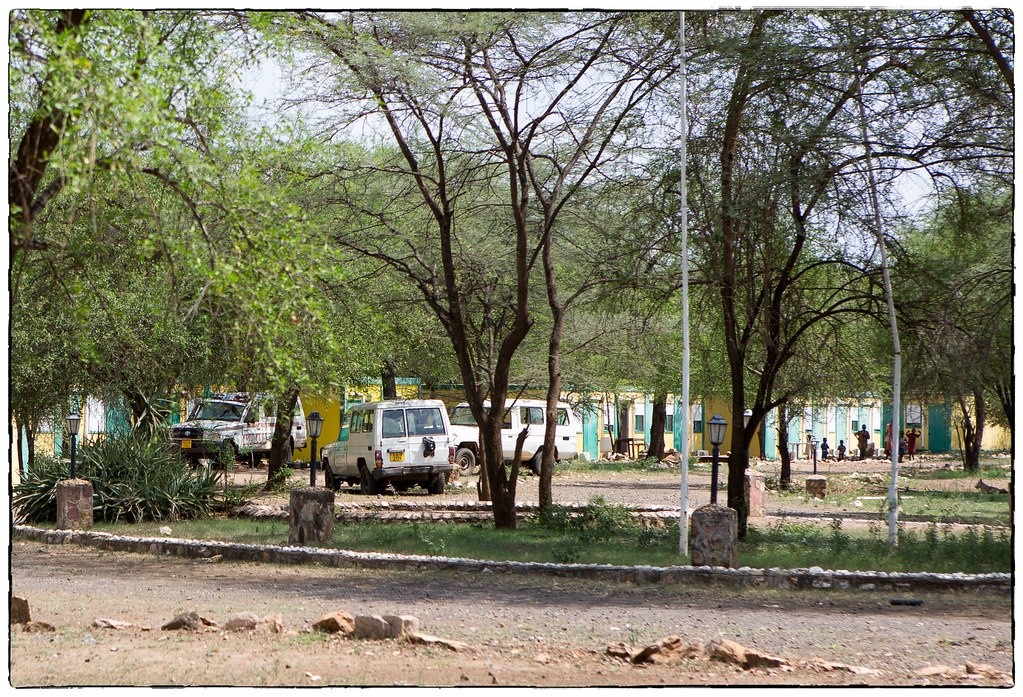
[
  {"xmin": 169, "ymin": 391, "xmax": 307, "ymax": 469},
  {"xmin": 324, "ymin": 398, "xmax": 454, "ymax": 494}
]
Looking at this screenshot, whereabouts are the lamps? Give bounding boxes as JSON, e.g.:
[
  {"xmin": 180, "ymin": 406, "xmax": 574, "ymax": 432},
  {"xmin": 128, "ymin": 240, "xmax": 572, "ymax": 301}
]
[
  {"xmin": 65, "ymin": 411, "xmax": 81, "ymax": 435},
  {"xmin": 809, "ymin": 435, "xmax": 819, "ymax": 451},
  {"xmin": 305, "ymin": 411, "xmax": 324, "ymax": 439},
  {"xmin": 705, "ymin": 415, "xmax": 729, "ymax": 448}
]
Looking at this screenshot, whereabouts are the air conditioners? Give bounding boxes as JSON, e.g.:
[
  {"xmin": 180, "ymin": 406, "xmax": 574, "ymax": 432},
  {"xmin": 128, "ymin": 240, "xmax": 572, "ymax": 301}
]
[
  {"xmin": 577, "ymin": 452, "xmax": 590, "ymax": 461},
  {"xmin": 697, "ymin": 450, "xmax": 709, "ymax": 457},
  {"xmin": 876, "ymin": 447, "xmax": 887, "ymax": 457}
]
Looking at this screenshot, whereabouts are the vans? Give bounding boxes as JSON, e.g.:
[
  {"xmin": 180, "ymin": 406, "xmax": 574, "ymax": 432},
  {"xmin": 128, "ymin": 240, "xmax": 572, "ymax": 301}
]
[{"xmin": 446, "ymin": 399, "xmax": 578, "ymax": 477}]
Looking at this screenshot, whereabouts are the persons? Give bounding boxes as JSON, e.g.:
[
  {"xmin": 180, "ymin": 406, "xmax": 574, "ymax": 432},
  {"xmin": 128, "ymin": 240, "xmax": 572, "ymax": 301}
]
[
  {"xmin": 854, "ymin": 424, "xmax": 870, "ymax": 460},
  {"xmin": 820, "ymin": 438, "xmax": 829, "ymax": 459},
  {"xmin": 837, "ymin": 440, "xmax": 846, "ymax": 462},
  {"xmin": 884, "ymin": 419, "xmax": 921, "ymax": 463}
]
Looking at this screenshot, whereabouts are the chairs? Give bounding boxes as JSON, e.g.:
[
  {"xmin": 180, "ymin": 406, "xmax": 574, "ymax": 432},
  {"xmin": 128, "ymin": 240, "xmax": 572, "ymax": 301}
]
[
  {"xmin": 382, "ymin": 417, "xmax": 401, "ymax": 434},
  {"xmin": 418, "ymin": 413, "xmax": 436, "ymax": 435}
]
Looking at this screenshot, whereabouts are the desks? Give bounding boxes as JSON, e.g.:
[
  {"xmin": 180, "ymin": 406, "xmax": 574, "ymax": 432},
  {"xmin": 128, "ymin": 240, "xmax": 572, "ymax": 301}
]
[{"xmin": 788, "ymin": 442, "xmax": 815, "ymax": 463}]
